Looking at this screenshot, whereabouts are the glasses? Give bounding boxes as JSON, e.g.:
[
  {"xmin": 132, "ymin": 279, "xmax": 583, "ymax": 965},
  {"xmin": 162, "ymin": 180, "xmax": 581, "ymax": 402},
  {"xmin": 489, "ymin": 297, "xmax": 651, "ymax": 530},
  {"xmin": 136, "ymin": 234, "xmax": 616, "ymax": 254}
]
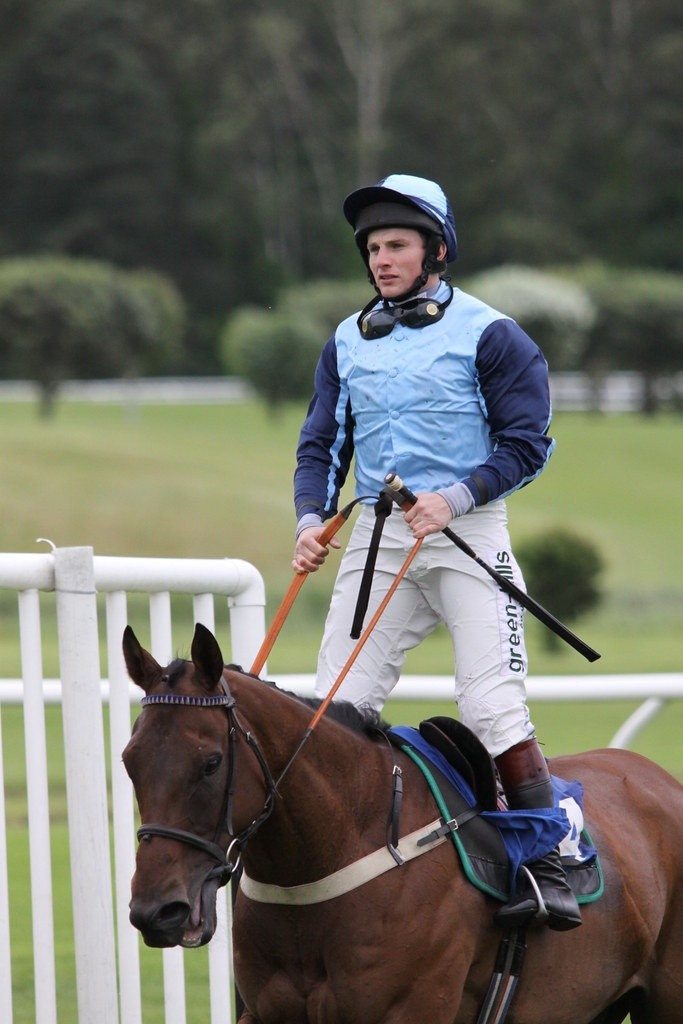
[{"xmin": 356, "ymin": 296, "xmax": 446, "ymax": 341}]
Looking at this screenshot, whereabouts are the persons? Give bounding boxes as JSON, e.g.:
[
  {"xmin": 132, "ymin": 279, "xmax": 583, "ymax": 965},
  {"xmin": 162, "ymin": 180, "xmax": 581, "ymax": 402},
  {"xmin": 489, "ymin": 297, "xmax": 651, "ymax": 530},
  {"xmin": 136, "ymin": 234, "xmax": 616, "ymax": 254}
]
[{"xmin": 293, "ymin": 174, "xmax": 583, "ymax": 933}]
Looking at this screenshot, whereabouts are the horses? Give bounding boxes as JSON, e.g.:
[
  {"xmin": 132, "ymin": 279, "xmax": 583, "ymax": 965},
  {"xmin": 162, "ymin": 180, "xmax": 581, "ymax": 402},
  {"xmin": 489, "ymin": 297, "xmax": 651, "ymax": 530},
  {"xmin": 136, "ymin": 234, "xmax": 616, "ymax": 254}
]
[{"xmin": 123, "ymin": 622, "xmax": 682, "ymax": 1024}]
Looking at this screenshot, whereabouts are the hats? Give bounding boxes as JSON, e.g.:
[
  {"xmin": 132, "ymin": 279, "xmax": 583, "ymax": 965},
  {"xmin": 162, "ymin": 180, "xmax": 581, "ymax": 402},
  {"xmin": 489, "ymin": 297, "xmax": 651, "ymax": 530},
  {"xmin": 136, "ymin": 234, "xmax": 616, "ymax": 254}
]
[{"xmin": 342, "ymin": 174, "xmax": 461, "ymax": 263}]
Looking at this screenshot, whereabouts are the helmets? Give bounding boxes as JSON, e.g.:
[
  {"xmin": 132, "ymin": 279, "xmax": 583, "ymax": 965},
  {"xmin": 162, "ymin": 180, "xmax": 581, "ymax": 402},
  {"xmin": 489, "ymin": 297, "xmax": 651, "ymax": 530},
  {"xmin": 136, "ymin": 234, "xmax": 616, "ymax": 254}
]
[{"xmin": 352, "ymin": 197, "xmax": 446, "ymax": 255}]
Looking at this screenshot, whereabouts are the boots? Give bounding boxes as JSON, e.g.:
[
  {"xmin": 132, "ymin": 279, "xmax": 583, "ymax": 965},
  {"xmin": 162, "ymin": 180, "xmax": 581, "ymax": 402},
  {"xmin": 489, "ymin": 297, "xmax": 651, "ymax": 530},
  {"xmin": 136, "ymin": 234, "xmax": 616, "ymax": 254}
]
[{"xmin": 491, "ymin": 735, "xmax": 583, "ymax": 932}]
